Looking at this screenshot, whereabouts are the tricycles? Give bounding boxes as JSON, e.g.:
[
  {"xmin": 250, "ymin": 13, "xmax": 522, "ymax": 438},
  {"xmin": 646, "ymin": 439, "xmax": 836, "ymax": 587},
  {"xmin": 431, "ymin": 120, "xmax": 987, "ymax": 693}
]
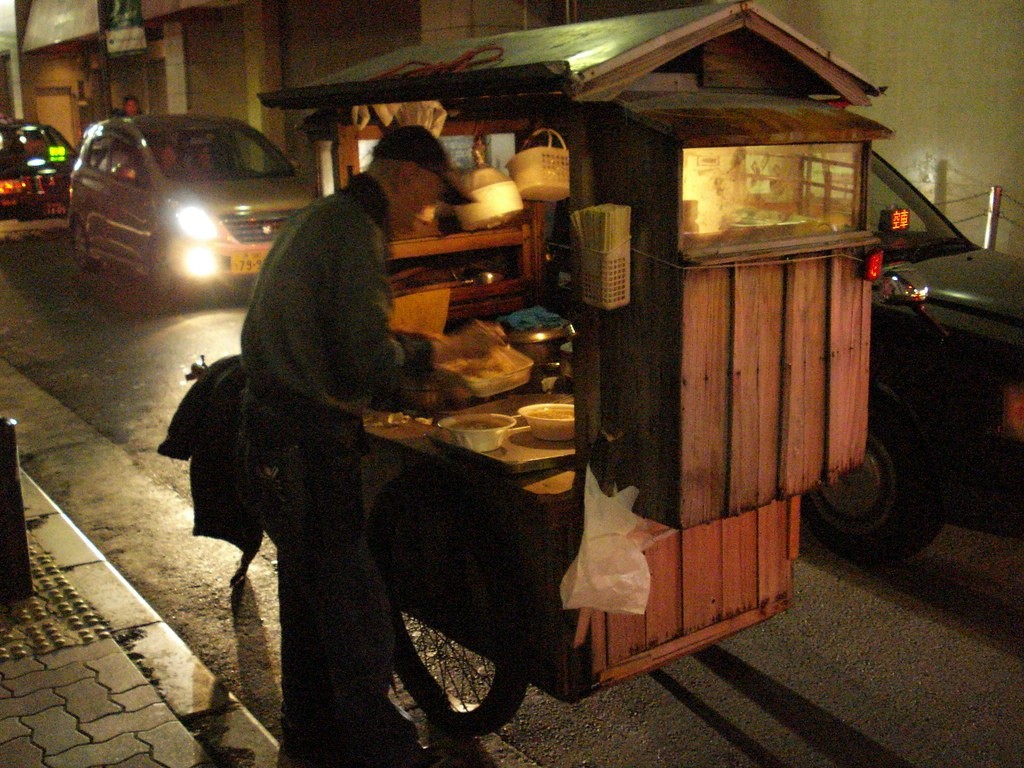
[{"xmin": 178, "ymin": 2, "xmax": 897, "ymax": 735}]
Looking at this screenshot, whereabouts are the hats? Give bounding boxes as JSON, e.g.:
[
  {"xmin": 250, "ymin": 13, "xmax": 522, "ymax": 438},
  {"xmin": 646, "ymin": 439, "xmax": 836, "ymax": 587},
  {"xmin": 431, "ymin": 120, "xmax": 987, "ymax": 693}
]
[{"xmin": 374, "ymin": 125, "xmax": 477, "ymax": 205}]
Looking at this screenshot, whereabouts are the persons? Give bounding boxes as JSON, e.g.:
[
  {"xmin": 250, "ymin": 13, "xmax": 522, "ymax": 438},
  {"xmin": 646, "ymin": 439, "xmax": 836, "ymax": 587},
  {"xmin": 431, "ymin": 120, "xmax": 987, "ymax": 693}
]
[
  {"xmin": 122, "ymin": 96, "xmax": 139, "ymax": 117},
  {"xmin": 239, "ymin": 125, "xmax": 507, "ymax": 768}
]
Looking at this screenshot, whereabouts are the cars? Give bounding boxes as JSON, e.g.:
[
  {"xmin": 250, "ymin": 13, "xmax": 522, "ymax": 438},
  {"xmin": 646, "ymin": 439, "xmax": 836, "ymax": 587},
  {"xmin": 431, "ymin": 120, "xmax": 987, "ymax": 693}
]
[
  {"xmin": 66, "ymin": 111, "xmax": 318, "ymax": 298},
  {"xmin": 800, "ymin": 145, "xmax": 1023, "ymax": 569},
  {"xmin": 0, "ymin": 115, "xmax": 79, "ymax": 221}
]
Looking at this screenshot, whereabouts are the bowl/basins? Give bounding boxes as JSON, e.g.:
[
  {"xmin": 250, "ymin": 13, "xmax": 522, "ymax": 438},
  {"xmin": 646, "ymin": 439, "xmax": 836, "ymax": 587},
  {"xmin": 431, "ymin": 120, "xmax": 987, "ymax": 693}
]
[
  {"xmin": 438, "ymin": 414, "xmax": 516, "ymax": 455},
  {"xmin": 518, "ymin": 404, "xmax": 576, "ymax": 441}
]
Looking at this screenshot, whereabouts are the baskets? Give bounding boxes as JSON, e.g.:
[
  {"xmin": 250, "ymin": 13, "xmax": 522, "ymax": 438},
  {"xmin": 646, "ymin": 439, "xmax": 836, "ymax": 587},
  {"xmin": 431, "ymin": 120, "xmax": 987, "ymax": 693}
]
[
  {"xmin": 582, "ymin": 234, "xmax": 631, "ymax": 310},
  {"xmin": 506, "ymin": 128, "xmax": 570, "ymax": 202}
]
